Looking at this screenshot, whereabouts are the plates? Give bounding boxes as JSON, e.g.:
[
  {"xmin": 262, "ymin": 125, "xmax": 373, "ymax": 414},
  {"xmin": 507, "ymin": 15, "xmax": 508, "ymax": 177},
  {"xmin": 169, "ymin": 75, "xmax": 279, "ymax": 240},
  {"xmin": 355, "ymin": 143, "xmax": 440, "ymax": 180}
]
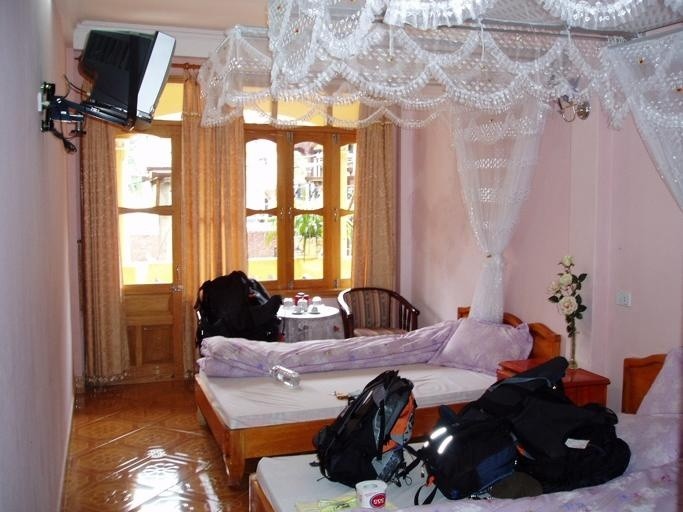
[
  {"xmin": 309, "ymin": 313, "xmax": 320, "ymax": 315},
  {"xmin": 292, "ymin": 313, "xmax": 303, "ymax": 315}
]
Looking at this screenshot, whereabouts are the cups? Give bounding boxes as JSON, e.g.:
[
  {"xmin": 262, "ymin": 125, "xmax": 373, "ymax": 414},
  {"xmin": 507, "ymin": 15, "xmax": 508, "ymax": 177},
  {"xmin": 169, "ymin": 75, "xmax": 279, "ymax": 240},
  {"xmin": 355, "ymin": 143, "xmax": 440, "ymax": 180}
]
[
  {"xmin": 312, "ymin": 296, "xmax": 321, "ymax": 312},
  {"xmin": 283, "ymin": 298, "xmax": 294, "ymax": 313}
]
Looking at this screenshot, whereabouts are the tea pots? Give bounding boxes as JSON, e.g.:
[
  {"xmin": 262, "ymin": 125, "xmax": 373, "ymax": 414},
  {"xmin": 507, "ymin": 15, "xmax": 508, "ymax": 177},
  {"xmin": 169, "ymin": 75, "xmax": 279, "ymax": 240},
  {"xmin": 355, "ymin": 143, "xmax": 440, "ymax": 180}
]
[{"xmin": 298, "ymin": 297, "xmax": 308, "ymax": 313}]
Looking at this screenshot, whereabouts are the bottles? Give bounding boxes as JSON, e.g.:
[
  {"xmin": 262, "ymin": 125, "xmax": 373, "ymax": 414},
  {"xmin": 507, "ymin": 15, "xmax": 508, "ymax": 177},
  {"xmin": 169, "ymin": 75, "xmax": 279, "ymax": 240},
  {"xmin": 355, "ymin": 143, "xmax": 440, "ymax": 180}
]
[{"xmin": 269, "ymin": 364, "xmax": 300, "ymax": 389}]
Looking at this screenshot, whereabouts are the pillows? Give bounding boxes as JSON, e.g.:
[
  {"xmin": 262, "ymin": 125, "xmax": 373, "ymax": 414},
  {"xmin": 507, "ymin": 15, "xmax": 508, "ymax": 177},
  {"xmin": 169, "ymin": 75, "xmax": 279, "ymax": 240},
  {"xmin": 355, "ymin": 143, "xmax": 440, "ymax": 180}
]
[{"xmin": 426, "ymin": 316, "xmax": 536, "ymax": 380}]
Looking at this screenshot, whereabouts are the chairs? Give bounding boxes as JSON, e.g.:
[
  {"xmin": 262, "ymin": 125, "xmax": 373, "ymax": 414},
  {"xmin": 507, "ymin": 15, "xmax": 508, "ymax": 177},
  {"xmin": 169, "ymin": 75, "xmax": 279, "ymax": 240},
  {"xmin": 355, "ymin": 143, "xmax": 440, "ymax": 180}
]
[{"xmin": 336, "ymin": 286, "xmax": 420, "ymax": 339}]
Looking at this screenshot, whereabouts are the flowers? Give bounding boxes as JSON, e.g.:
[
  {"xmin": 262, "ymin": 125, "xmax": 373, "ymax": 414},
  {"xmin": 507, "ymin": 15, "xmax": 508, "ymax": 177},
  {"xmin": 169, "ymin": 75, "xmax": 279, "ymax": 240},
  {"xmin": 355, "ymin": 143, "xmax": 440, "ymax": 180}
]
[{"xmin": 545, "ymin": 253, "xmax": 588, "ymax": 368}]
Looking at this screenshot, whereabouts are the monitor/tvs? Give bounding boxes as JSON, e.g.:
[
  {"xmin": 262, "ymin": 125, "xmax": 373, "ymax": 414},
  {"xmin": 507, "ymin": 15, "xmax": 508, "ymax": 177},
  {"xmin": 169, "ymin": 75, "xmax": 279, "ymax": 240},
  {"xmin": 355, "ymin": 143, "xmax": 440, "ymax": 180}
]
[{"xmin": 77, "ymin": 28, "xmax": 177, "ymax": 131}]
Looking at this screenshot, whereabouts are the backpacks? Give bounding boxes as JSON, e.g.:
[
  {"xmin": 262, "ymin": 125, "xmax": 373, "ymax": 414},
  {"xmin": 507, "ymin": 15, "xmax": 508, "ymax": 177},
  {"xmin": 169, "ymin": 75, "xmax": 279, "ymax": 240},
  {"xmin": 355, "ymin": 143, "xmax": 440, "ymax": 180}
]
[
  {"xmin": 412, "ymin": 356, "xmax": 631, "ymax": 506},
  {"xmin": 314, "ymin": 369, "xmax": 418, "ymax": 488}
]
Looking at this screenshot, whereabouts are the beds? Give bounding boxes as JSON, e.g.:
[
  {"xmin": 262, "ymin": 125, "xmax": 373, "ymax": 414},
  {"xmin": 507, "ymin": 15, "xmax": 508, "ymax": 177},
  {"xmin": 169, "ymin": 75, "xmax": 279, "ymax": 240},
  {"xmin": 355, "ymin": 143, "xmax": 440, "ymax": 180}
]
[
  {"xmin": 246, "ymin": 350, "xmax": 682, "ymax": 510},
  {"xmin": 195, "ymin": 304, "xmax": 564, "ymax": 488}
]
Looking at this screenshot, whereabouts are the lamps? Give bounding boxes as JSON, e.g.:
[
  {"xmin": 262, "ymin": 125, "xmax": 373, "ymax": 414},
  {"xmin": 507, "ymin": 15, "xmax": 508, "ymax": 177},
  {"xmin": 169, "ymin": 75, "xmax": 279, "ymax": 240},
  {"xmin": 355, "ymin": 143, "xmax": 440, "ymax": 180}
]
[{"xmin": 544, "ymin": 74, "xmax": 590, "ymax": 124}]
